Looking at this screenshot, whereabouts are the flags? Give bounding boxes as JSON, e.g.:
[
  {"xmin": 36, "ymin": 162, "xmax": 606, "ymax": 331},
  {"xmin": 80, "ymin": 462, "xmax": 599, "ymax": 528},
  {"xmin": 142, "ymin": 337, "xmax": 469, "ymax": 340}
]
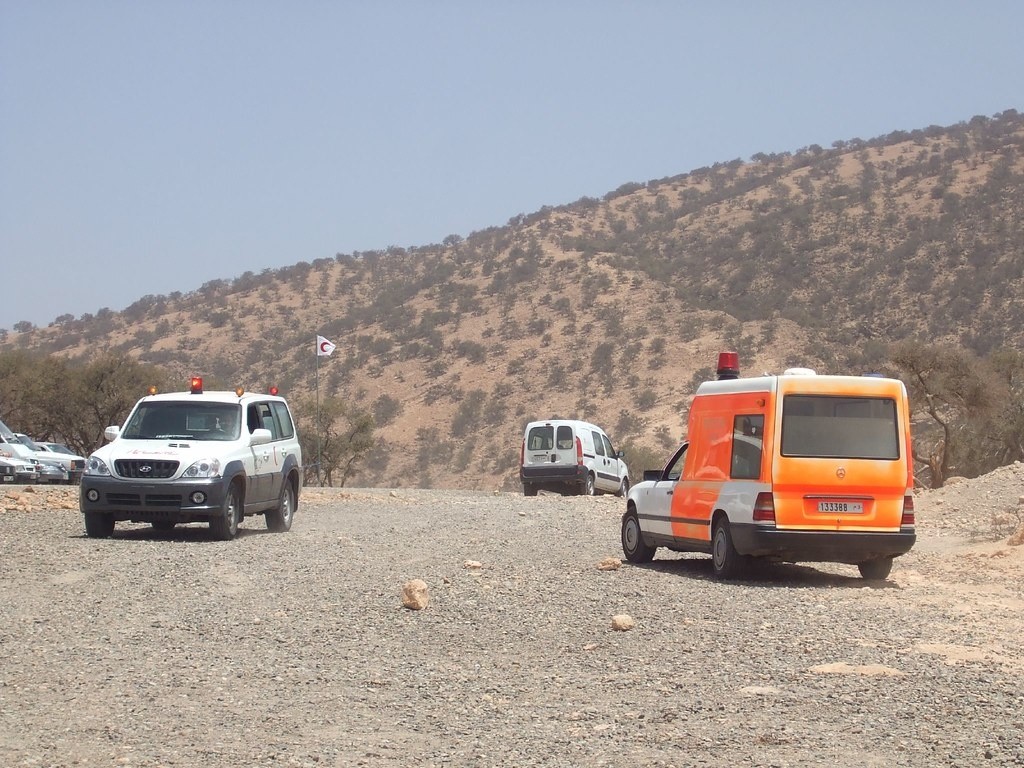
[{"xmin": 317, "ymin": 335, "xmax": 336, "ymax": 357}]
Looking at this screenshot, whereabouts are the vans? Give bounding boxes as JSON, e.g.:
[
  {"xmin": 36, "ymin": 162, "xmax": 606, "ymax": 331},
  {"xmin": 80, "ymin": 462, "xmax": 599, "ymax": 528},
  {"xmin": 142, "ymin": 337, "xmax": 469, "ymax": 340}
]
[{"xmin": 520, "ymin": 420, "xmax": 631, "ymax": 498}]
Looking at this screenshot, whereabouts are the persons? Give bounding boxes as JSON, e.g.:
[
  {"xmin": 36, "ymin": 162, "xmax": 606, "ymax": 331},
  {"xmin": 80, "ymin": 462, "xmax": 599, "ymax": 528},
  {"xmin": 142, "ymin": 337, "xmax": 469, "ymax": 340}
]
[{"xmin": 218, "ymin": 411, "xmax": 235, "ymax": 433}]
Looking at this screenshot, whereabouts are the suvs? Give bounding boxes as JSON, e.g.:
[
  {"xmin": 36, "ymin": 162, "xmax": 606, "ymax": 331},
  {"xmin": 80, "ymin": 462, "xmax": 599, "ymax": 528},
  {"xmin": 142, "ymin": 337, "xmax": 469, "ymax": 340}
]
[
  {"xmin": 79, "ymin": 377, "xmax": 304, "ymax": 540},
  {"xmin": 0, "ymin": 420, "xmax": 89, "ymax": 485}
]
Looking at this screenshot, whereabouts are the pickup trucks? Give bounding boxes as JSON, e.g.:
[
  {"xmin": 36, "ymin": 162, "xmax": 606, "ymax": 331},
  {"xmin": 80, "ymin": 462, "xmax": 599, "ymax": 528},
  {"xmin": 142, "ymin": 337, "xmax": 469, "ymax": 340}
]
[{"xmin": 621, "ymin": 352, "xmax": 916, "ymax": 580}]
